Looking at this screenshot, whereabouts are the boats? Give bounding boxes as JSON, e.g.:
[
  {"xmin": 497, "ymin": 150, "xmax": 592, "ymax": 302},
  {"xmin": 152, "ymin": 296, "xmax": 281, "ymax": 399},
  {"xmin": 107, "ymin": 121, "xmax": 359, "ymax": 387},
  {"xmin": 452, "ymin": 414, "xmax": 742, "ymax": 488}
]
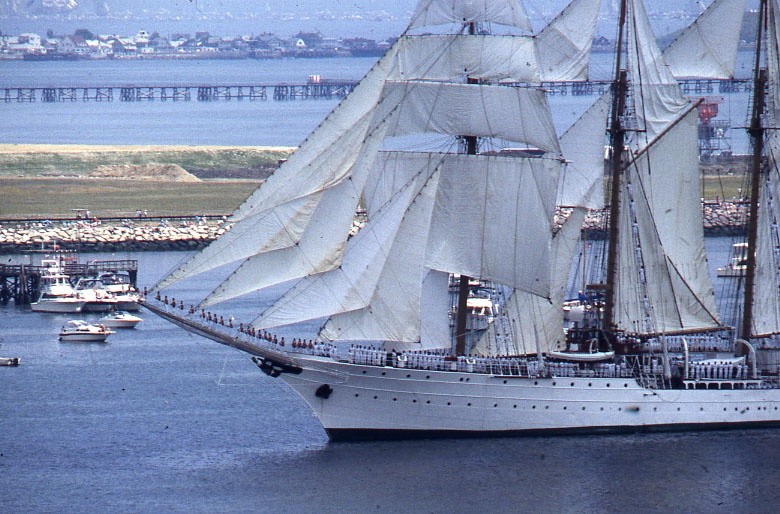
[
  {"xmin": 59, "ymin": 314, "xmax": 116, "ymax": 341},
  {"xmin": 93, "ymin": 270, "xmax": 142, "ymax": 311},
  {"xmin": 447, "ymin": 273, "xmax": 502, "ymax": 332},
  {"xmin": 98, "ymin": 302, "xmax": 142, "ymax": 328},
  {"xmin": 30, "ymin": 253, "xmax": 89, "ymax": 314},
  {"xmin": 70, "ymin": 276, "xmax": 116, "ymax": 313},
  {"xmin": 562, "ymin": 283, "xmax": 607, "ymax": 322},
  {"xmin": 717, "ymin": 243, "xmax": 755, "ymax": 278}
]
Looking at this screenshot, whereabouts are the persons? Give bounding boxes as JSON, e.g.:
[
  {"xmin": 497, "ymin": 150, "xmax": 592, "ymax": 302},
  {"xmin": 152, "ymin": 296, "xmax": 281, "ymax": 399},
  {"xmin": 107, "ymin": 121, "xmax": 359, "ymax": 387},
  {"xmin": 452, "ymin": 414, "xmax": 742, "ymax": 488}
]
[
  {"xmin": 144, "ymin": 287, "xmax": 747, "ymax": 396},
  {"xmin": 13, "ymin": 211, "xmax": 229, "ymax": 229}
]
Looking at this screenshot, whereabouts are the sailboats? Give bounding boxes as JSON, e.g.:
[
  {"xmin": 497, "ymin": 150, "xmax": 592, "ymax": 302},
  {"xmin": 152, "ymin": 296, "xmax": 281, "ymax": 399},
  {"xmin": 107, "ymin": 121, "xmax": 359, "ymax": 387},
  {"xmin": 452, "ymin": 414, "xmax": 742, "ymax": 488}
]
[
  {"xmin": 138, "ymin": 0, "xmax": 780, "ymax": 444},
  {"xmin": 542, "ymin": 229, "xmax": 615, "ymax": 363}
]
